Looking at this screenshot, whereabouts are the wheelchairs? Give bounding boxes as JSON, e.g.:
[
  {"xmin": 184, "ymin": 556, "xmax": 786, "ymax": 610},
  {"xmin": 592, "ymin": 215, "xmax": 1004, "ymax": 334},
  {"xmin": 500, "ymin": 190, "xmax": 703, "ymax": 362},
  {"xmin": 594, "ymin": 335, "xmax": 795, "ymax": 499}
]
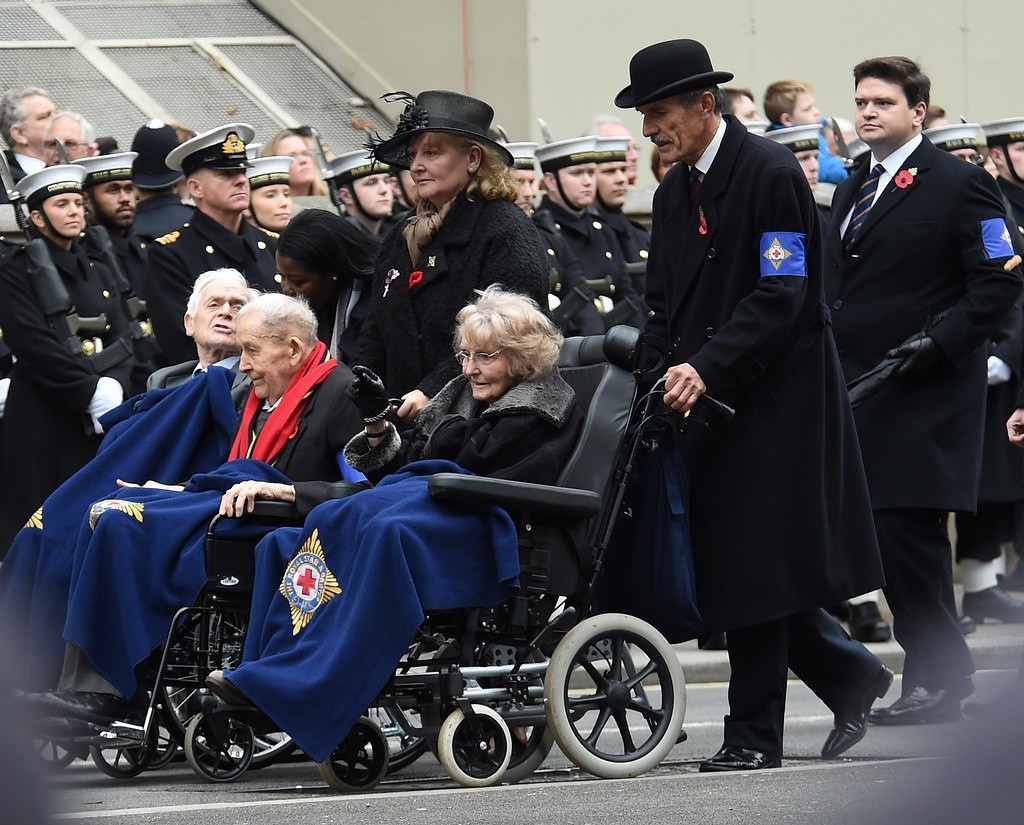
[{"xmin": 1, "ymin": 325, "xmax": 737, "ymax": 795}]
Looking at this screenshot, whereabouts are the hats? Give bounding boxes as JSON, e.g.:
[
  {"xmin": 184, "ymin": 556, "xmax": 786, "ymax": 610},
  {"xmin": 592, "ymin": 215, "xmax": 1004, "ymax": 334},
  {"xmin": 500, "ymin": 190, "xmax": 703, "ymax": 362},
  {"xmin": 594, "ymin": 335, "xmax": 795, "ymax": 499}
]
[
  {"xmin": 362, "ymin": 90, "xmax": 514, "ymax": 171},
  {"xmin": 165, "ymin": 123, "xmax": 255, "ymax": 178},
  {"xmin": 536, "ymin": 135, "xmax": 596, "ymax": 173},
  {"xmin": 329, "ymin": 149, "xmax": 390, "ymax": 186},
  {"xmin": 246, "ymin": 156, "xmax": 294, "ymax": 190},
  {"xmin": 595, "ymin": 137, "xmax": 631, "ymax": 163},
  {"xmin": 923, "ymin": 123, "xmax": 980, "ymax": 151},
  {"xmin": 505, "ymin": 142, "xmax": 539, "ymax": 169},
  {"xmin": 131, "ymin": 120, "xmax": 183, "ymax": 188},
  {"xmin": 615, "ymin": 40, "xmax": 735, "ymax": 109},
  {"xmin": 762, "ymin": 122, "xmax": 823, "ymax": 151},
  {"xmin": 15, "ymin": 165, "xmax": 87, "ymax": 213},
  {"xmin": 982, "ymin": 117, "xmax": 1024, "ymax": 145},
  {"xmin": 70, "ymin": 151, "xmax": 140, "ymax": 186}
]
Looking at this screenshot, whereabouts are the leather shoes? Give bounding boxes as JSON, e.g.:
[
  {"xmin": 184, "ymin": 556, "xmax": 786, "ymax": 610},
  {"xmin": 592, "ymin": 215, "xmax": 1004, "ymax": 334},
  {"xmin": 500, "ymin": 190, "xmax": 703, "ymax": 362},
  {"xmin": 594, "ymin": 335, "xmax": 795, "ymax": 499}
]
[
  {"xmin": 698, "ymin": 748, "xmax": 783, "ymax": 771},
  {"xmin": 868, "ymin": 678, "xmax": 975, "ymax": 725},
  {"xmin": 821, "ymin": 665, "xmax": 894, "ymax": 760}
]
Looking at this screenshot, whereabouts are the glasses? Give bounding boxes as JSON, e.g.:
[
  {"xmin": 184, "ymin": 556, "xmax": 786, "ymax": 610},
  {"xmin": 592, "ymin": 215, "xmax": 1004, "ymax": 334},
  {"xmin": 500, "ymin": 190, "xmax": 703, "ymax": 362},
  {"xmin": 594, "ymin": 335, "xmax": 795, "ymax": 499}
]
[
  {"xmin": 455, "ymin": 345, "xmax": 509, "ymax": 366},
  {"xmin": 45, "ymin": 139, "xmax": 87, "ymax": 150}
]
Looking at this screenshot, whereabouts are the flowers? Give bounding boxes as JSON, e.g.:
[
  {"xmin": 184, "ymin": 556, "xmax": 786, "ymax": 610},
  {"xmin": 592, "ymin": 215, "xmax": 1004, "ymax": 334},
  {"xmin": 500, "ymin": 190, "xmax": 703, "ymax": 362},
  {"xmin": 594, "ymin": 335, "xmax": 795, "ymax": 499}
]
[
  {"xmin": 363, "ymin": 91, "xmax": 429, "ymax": 171},
  {"xmin": 699, "ymin": 206, "xmax": 707, "ymax": 235},
  {"xmin": 891, "ymin": 171, "xmax": 914, "ymax": 193}
]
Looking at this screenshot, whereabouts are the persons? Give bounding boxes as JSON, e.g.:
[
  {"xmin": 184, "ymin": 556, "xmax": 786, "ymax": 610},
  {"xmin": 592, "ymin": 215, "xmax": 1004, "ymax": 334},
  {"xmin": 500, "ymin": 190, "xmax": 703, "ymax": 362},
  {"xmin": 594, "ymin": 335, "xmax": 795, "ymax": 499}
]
[
  {"xmin": 0, "ymin": 38, "xmax": 1024, "ymax": 764},
  {"xmin": 823, "ymin": 55, "xmax": 1024, "ymax": 727},
  {"xmin": 206, "ymin": 281, "xmax": 586, "ymax": 767},
  {"xmin": 616, "ymin": 40, "xmax": 894, "ymax": 770}
]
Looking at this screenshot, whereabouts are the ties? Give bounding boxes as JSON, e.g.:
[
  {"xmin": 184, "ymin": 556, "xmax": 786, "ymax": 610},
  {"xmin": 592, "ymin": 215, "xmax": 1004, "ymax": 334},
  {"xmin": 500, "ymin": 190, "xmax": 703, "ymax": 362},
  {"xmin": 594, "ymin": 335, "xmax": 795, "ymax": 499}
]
[{"xmin": 841, "ymin": 165, "xmax": 885, "ymax": 242}]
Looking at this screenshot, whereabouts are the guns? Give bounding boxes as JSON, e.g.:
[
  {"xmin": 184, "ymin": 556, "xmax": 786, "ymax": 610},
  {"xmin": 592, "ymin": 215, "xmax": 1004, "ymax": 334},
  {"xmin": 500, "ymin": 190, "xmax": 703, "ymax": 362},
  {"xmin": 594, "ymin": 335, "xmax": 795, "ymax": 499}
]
[
  {"xmin": 537, "ymin": 117, "xmax": 646, "ymax": 276},
  {"xmin": 496, "ymin": 123, "xmax": 640, "ymax": 333},
  {"xmin": 310, "ymin": 126, "xmax": 362, "ymax": 232},
  {"xmin": 53, "ymin": 137, "xmax": 163, "ymax": 360},
  {"xmin": 549, "ymin": 264, "xmax": 591, "ymax": 337},
  {"xmin": 0, "ymin": 152, "xmax": 131, "ymax": 436}
]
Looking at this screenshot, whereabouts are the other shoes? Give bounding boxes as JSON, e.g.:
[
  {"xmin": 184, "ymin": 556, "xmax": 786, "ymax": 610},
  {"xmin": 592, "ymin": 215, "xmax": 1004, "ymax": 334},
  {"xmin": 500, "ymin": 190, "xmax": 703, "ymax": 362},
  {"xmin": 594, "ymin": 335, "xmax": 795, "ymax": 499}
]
[
  {"xmin": 963, "ymin": 585, "xmax": 1024, "ymax": 624},
  {"xmin": 29, "ymin": 688, "xmax": 128, "ymax": 717},
  {"xmin": 203, "ymin": 670, "xmax": 247, "ymax": 705},
  {"xmin": 959, "ymin": 615, "xmax": 977, "ymax": 633},
  {"xmin": 850, "ymin": 602, "xmax": 893, "ymax": 641}
]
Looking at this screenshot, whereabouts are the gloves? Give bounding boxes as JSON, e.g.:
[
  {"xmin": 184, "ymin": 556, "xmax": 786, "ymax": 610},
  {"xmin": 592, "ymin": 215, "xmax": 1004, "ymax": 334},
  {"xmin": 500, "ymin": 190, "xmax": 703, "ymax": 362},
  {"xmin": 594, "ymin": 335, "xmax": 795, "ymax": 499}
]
[
  {"xmin": 884, "ymin": 336, "xmax": 935, "ymax": 377},
  {"xmin": 0, "ymin": 377, "xmax": 12, "ymax": 419},
  {"xmin": 87, "ymin": 378, "xmax": 123, "ymax": 434},
  {"xmin": 986, "ymin": 356, "xmax": 1013, "ymax": 387},
  {"xmin": 344, "ymin": 366, "xmax": 392, "ymax": 426}
]
